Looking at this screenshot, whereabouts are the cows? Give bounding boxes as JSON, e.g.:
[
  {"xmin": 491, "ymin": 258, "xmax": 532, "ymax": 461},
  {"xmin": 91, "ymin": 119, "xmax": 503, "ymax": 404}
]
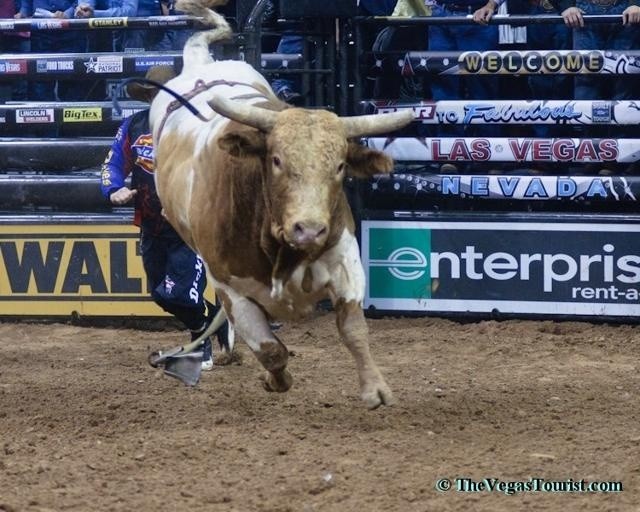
[{"xmin": 149, "ymin": 0, "xmax": 415, "ymax": 410}]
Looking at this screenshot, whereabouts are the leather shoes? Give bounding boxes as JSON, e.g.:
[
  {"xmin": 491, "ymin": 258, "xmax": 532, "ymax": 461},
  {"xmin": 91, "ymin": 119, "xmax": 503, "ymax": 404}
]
[{"xmin": 280, "ymin": 89, "xmax": 304, "ymax": 106}]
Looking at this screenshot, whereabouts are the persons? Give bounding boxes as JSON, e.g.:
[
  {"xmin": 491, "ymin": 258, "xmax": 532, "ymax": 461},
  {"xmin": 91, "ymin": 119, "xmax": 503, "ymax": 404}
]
[
  {"xmin": 0, "ymin": 0, "xmax": 640, "ymax": 139},
  {"xmin": 101, "ymin": 64, "xmax": 235, "ymax": 372}
]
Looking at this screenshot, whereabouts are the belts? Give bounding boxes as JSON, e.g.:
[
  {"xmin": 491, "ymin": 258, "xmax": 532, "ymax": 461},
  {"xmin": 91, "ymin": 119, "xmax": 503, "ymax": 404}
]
[
  {"xmin": 591, "ymin": 0, "xmax": 620, "ymax": 9},
  {"xmin": 437, "ymin": 1, "xmax": 481, "ymax": 11},
  {"xmin": 529, "ymin": 1, "xmax": 557, "ymax": 13}
]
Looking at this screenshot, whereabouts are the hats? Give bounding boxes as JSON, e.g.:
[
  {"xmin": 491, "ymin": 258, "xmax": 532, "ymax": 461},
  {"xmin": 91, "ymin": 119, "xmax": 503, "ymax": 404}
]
[{"xmin": 126, "ymin": 66, "xmax": 176, "ymax": 101}]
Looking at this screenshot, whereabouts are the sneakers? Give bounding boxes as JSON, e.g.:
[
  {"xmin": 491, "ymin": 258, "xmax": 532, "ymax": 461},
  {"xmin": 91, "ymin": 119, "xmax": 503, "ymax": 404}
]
[
  {"xmin": 190, "ymin": 331, "xmax": 213, "ymax": 371},
  {"xmin": 216, "ymin": 319, "xmax": 235, "ymax": 359}
]
